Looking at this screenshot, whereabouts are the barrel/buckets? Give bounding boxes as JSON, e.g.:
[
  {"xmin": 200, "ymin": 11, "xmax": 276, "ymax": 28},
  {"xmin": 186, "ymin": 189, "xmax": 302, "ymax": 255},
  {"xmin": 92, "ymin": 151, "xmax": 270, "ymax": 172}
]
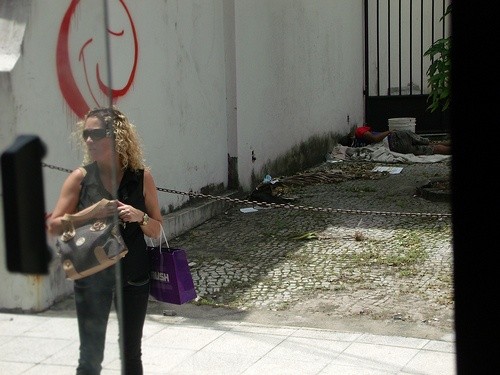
[{"xmin": 388, "ymin": 117, "xmax": 416, "ymax": 154}]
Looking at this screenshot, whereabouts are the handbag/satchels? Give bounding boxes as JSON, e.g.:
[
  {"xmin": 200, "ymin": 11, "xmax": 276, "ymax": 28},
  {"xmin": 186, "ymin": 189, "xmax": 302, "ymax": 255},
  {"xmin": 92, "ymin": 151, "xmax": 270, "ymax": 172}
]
[
  {"xmin": 56, "ymin": 167, "xmax": 129, "ymax": 280},
  {"xmin": 148, "ymin": 221, "xmax": 197, "ymax": 305}
]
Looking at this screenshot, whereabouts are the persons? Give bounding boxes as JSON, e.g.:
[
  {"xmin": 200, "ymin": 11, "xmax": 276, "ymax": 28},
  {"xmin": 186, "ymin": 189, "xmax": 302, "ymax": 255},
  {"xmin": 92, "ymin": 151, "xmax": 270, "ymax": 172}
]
[
  {"xmin": 340, "ymin": 127, "xmax": 450, "ymax": 155},
  {"xmin": 47, "ymin": 106, "xmax": 163, "ymax": 375}
]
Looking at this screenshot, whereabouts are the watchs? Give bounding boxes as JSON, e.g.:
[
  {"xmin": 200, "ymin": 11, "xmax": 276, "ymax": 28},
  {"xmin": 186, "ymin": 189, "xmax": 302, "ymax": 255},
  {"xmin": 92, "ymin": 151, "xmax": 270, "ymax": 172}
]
[{"xmin": 137, "ymin": 212, "xmax": 150, "ymax": 226}]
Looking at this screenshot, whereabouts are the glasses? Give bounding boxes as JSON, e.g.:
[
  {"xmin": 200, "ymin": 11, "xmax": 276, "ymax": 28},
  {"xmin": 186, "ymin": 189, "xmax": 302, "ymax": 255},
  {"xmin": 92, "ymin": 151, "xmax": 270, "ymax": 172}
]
[{"xmin": 83, "ymin": 128, "xmax": 107, "ymax": 141}]
